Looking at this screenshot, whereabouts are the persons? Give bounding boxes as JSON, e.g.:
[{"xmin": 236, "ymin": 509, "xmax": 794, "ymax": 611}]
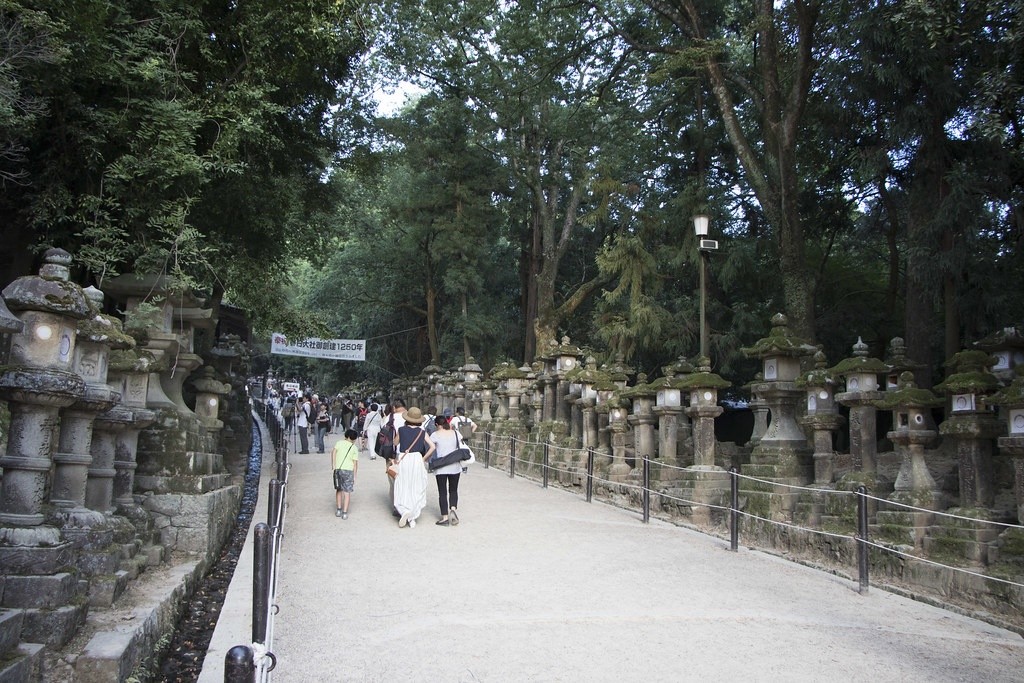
[
  {"xmin": 392, "ymin": 407, "xmax": 435, "ymax": 530},
  {"xmin": 430, "ymin": 416, "xmax": 467, "ymax": 525},
  {"xmin": 382, "ymin": 398, "xmax": 409, "ymax": 518},
  {"xmin": 331, "ymin": 429, "xmax": 358, "ymax": 520},
  {"xmin": 267, "ymin": 379, "xmax": 477, "ymax": 474}
]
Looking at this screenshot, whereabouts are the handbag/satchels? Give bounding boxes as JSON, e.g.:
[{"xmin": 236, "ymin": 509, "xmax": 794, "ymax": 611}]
[
  {"xmin": 387, "ymin": 466, "xmax": 397, "ymax": 478},
  {"xmin": 458, "ymin": 415, "xmax": 472, "ymax": 439},
  {"xmin": 364, "ymin": 430, "xmax": 367, "ymax": 438},
  {"xmin": 333, "ymin": 469, "xmax": 340, "ymax": 489}
]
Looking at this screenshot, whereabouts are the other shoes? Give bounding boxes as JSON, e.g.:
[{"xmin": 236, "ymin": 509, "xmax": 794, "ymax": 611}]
[
  {"xmin": 342, "ymin": 512, "xmax": 348, "ymax": 520},
  {"xmin": 409, "ymin": 519, "xmax": 416, "ymax": 528},
  {"xmin": 335, "ymin": 509, "xmax": 341, "ymax": 517},
  {"xmin": 398, "ymin": 510, "xmax": 410, "ymax": 528},
  {"xmin": 450, "ymin": 510, "xmax": 459, "ymax": 526},
  {"xmin": 462, "ymin": 467, "xmax": 467, "ymax": 472},
  {"xmin": 317, "ymin": 449, "xmax": 324, "ymax": 454},
  {"xmin": 436, "ymin": 520, "xmax": 449, "ymax": 526},
  {"xmin": 299, "ymin": 450, "xmax": 310, "ymax": 454}
]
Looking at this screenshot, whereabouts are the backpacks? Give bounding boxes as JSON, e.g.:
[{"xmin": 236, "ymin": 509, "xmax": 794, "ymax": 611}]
[
  {"xmin": 358, "ymin": 408, "xmax": 368, "ymax": 425},
  {"xmin": 303, "ymin": 403, "xmax": 317, "ymax": 424},
  {"xmin": 425, "ymin": 414, "xmax": 438, "ymax": 436},
  {"xmin": 323, "ymin": 412, "xmax": 332, "ymax": 432},
  {"xmin": 374, "ymin": 414, "xmax": 396, "ymax": 459}
]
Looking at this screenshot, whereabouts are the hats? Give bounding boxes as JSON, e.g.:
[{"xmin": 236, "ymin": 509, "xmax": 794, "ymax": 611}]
[
  {"xmin": 444, "ymin": 408, "xmax": 453, "ymax": 416},
  {"xmin": 402, "ymin": 407, "xmax": 426, "ymax": 424}
]
